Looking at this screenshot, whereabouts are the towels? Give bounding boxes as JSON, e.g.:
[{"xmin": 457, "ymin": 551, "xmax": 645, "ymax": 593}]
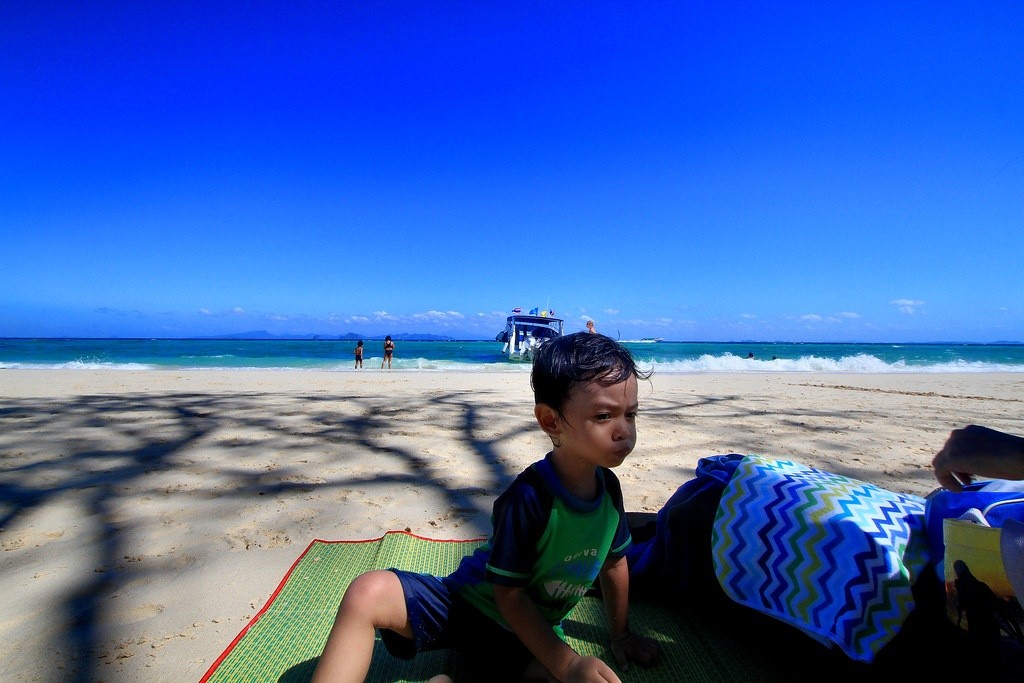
[{"xmin": 711, "ymin": 455, "xmax": 927, "ymax": 661}]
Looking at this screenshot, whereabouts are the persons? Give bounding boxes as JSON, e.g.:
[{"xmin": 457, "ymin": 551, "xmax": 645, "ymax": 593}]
[
  {"xmin": 381, "ymin": 336, "xmax": 394, "ymax": 369},
  {"xmin": 310, "ymin": 332, "xmax": 663, "ymax": 683},
  {"xmin": 932, "ymin": 425, "xmax": 1024, "ymax": 492},
  {"xmin": 586, "ymin": 321, "xmax": 595, "ymax": 334},
  {"xmin": 354, "ymin": 340, "xmax": 363, "ymax": 369}
]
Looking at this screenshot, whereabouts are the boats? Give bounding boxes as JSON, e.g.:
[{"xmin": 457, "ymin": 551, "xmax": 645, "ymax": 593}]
[
  {"xmin": 496, "ymin": 314, "xmax": 564, "ymax": 362},
  {"xmin": 641, "ymin": 338, "xmax": 665, "ymax": 343}
]
